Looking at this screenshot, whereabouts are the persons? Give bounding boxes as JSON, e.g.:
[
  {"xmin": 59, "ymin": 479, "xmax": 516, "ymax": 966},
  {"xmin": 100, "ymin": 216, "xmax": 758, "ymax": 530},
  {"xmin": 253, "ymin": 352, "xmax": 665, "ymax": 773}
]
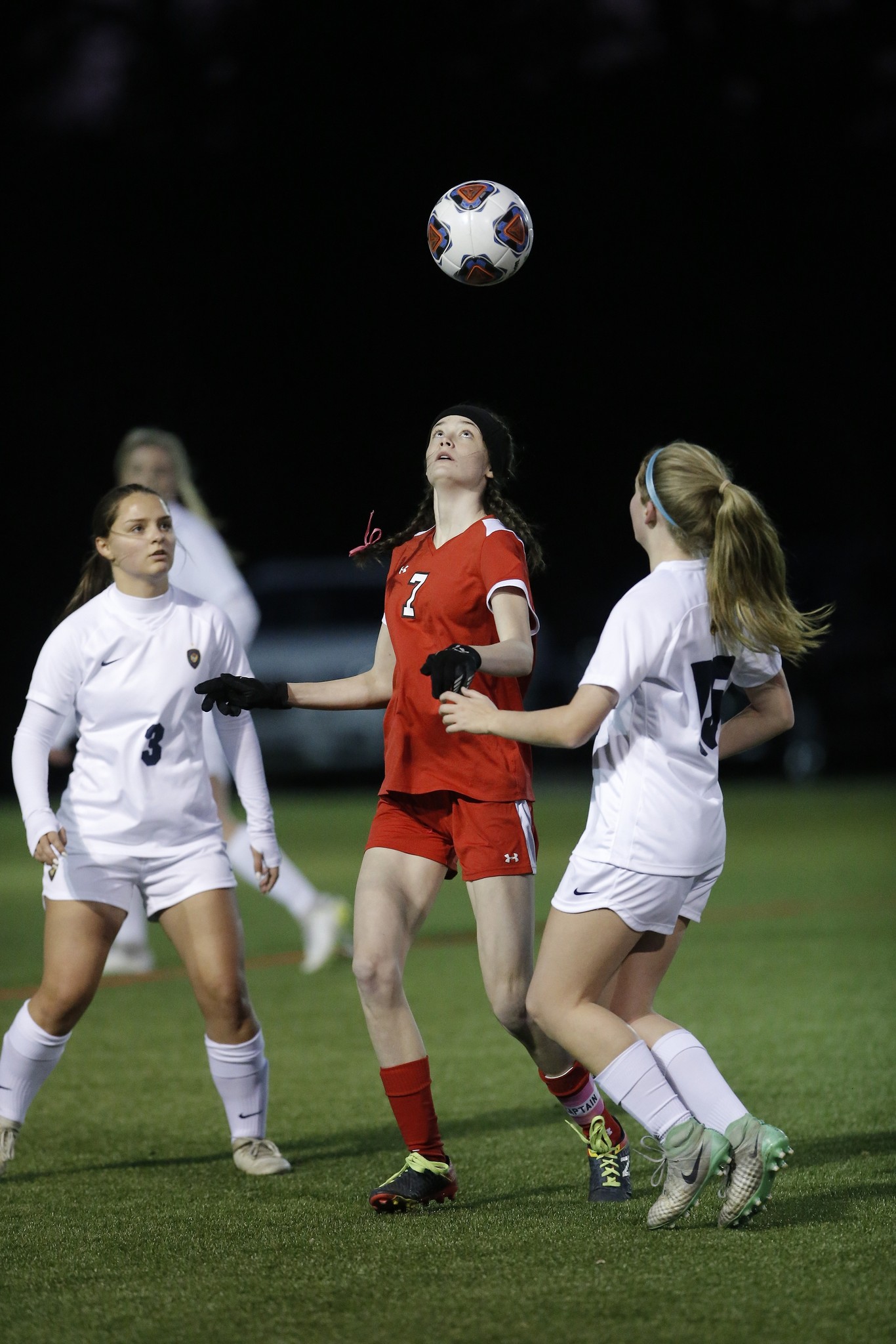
[
  {"xmin": 440, "ymin": 442, "xmax": 839, "ymax": 1236},
  {"xmin": 193, "ymin": 401, "xmax": 638, "ymax": 1216},
  {"xmin": 93, "ymin": 425, "xmax": 351, "ymax": 983},
  {"xmin": 0, "ymin": 482, "xmax": 292, "ymax": 1175}
]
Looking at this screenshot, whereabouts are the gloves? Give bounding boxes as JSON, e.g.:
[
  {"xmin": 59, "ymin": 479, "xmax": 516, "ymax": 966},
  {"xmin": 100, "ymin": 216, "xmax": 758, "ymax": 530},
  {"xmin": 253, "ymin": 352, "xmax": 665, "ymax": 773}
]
[
  {"xmin": 194, "ymin": 673, "xmax": 292, "ymax": 717},
  {"xmin": 420, "ymin": 643, "xmax": 482, "ymax": 700}
]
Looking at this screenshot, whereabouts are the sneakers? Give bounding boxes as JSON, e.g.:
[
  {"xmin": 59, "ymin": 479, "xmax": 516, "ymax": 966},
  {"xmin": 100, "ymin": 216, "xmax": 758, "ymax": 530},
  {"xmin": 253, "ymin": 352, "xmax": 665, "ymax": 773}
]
[
  {"xmin": 716, "ymin": 1113, "xmax": 794, "ymax": 1230},
  {"xmin": 0, "ymin": 1115, "xmax": 23, "ymax": 1179},
  {"xmin": 100, "ymin": 950, "xmax": 154, "ymax": 977},
  {"xmin": 646, "ymin": 1116, "xmax": 733, "ymax": 1230},
  {"xmin": 232, "ymin": 1137, "xmax": 293, "ymax": 1176},
  {"xmin": 368, "ymin": 1151, "xmax": 459, "ymax": 1214},
  {"xmin": 301, "ymin": 895, "xmax": 351, "ymax": 974},
  {"xmin": 587, "ymin": 1114, "xmax": 632, "ymax": 1202}
]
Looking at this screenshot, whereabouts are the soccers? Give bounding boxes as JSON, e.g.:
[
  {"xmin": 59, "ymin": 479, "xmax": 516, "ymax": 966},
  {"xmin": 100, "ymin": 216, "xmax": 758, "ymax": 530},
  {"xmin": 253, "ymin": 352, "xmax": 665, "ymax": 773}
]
[{"xmin": 427, "ymin": 179, "xmax": 533, "ymax": 288}]
